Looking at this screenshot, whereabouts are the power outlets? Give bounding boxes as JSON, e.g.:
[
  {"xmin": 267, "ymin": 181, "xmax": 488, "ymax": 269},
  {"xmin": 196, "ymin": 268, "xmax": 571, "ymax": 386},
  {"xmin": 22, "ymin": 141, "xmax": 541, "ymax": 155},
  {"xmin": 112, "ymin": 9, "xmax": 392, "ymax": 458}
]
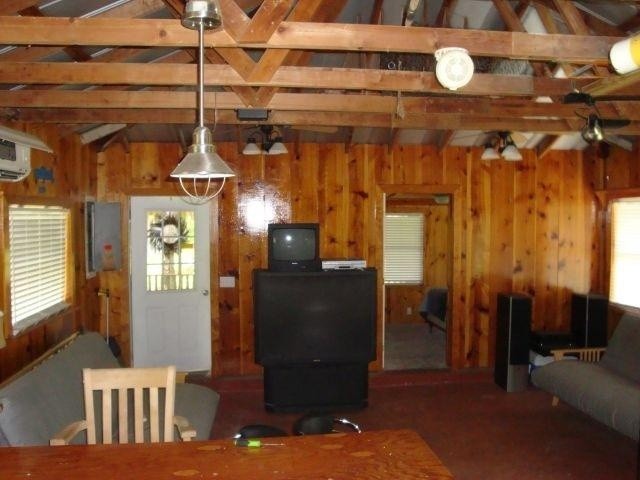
[{"xmin": 219, "ymin": 276, "xmax": 235, "ymax": 287}]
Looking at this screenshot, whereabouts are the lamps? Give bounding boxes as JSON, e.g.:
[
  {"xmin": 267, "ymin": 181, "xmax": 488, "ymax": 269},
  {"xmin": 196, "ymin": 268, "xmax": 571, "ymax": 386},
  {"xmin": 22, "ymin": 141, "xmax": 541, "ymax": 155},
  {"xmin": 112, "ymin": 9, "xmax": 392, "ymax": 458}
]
[
  {"xmin": 480, "ymin": 132, "xmax": 523, "ymax": 161},
  {"xmin": 169, "ymin": 0, "xmax": 236, "ymax": 202},
  {"xmin": 240, "ymin": 125, "xmax": 288, "ymax": 155}
]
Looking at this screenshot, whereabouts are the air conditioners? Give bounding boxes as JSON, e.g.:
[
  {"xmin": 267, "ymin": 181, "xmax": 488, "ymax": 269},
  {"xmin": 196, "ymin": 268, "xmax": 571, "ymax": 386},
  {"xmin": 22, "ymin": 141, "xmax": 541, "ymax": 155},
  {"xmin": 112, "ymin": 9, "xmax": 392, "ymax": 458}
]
[{"xmin": 0, "ymin": 124, "xmax": 53, "ymax": 183}]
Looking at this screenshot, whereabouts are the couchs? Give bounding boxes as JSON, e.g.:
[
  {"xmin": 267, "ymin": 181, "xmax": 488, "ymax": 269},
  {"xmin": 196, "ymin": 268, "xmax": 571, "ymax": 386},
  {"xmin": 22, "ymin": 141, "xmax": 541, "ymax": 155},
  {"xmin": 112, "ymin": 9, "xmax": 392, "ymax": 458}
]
[
  {"xmin": 528, "ymin": 309, "xmax": 639, "ymax": 437},
  {"xmin": 1, "ymin": 328, "xmax": 220, "ymax": 445}
]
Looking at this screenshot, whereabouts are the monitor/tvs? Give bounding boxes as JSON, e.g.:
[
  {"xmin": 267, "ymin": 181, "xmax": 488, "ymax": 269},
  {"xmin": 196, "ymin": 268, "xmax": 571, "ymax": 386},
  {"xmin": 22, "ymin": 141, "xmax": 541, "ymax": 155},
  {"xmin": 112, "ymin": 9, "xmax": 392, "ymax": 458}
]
[
  {"xmin": 250, "ymin": 268, "xmax": 377, "ymax": 365},
  {"xmin": 268, "ymin": 224, "xmax": 321, "ymax": 272}
]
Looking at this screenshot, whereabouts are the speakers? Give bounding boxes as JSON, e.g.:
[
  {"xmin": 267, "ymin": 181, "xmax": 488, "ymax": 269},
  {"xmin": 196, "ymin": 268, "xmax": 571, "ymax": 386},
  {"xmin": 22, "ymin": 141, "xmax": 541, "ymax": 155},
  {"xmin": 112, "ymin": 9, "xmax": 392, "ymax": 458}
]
[
  {"xmin": 571, "ymin": 294, "xmax": 608, "ymax": 349},
  {"xmin": 494, "ymin": 292, "xmax": 532, "ymax": 393}
]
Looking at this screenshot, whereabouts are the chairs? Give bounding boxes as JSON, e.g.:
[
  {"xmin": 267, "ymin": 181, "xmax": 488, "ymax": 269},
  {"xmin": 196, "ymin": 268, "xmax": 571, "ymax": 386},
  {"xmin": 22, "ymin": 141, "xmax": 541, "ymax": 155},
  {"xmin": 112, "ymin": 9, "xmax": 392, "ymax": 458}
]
[{"xmin": 48, "ymin": 364, "xmax": 198, "ymax": 445}]
